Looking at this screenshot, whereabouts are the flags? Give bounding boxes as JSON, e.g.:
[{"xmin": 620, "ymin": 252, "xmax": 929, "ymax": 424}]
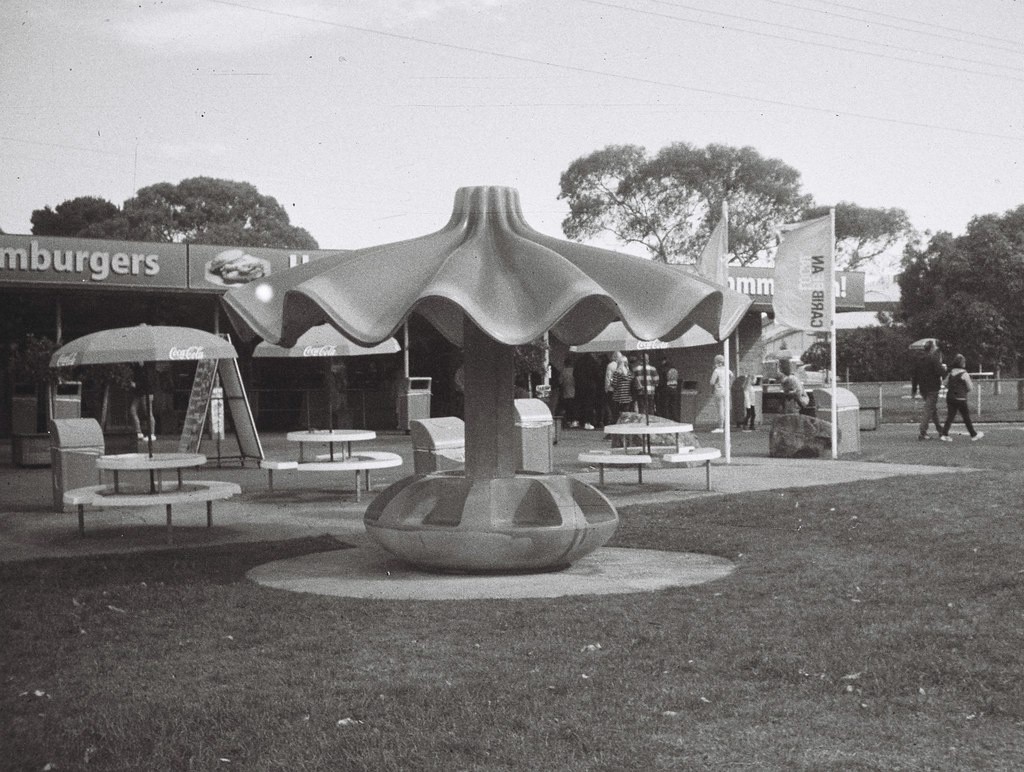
[
  {"xmin": 772, "ymin": 216, "xmax": 830, "ymax": 333},
  {"xmin": 698, "ymin": 217, "xmax": 723, "ymax": 284}
]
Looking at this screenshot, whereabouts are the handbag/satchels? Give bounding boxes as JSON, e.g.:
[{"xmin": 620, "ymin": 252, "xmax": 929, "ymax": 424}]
[{"xmin": 630, "ymin": 376, "xmax": 643, "ymax": 395}]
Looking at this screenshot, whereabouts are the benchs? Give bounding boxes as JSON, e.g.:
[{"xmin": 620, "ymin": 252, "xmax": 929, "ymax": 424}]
[
  {"xmin": 578, "ymin": 445, "xmax": 722, "ymax": 492},
  {"xmin": 63, "ymin": 482, "xmax": 242, "ymax": 545},
  {"xmin": 260, "ymin": 450, "xmax": 402, "ymax": 501}
]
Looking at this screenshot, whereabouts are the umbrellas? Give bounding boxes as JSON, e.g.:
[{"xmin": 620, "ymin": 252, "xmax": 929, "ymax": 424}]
[
  {"xmin": 46, "ymin": 321, "xmax": 242, "ymax": 493},
  {"xmin": 908, "ymin": 337, "xmax": 949, "ymax": 352},
  {"xmin": 221, "ymin": 185, "xmax": 754, "ymax": 476},
  {"xmin": 251, "ymin": 322, "xmax": 402, "ymax": 462}
]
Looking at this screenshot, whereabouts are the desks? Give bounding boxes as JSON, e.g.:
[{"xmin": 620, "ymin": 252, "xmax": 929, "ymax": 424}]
[
  {"xmin": 604, "ymin": 422, "xmax": 694, "ymax": 454},
  {"xmin": 95, "ymin": 452, "xmax": 207, "ymax": 494},
  {"xmin": 287, "ymin": 429, "xmax": 377, "ymax": 462}
]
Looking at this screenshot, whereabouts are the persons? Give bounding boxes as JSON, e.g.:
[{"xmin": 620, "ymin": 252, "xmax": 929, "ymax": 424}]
[
  {"xmin": 557, "ymin": 351, "xmax": 764, "ymax": 433},
  {"xmin": 912, "ymin": 341, "xmax": 948, "ymax": 440},
  {"xmin": 775, "ymin": 360, "xmax": 809, "ymax": 413},
  {"xmin": 940, "ymin": 354, "xmax": 984, "ymax": 442},
  {"xmin": 129, "ymin": 362, "xmax": 157, "ymax": 441}
]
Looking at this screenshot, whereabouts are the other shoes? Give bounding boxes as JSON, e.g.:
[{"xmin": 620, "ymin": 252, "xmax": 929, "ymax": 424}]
[
  {"xmin": 940, "ymin": 435, "xmax": 954, "ymax": 441},
  {"xmin": 143, "ymin": 434, "xmax": 156, "ymax": 442},
  {"xmin": 570, "ymin": 421, "xmax": 578, "ymax": 427},
  {"xmin": 137, "ymin": 432, "xmax": 144, "ymax": 439},
  {"xmin": 711, "ymin": 428, "xmax": 723, "ymax": 434},
  {"xmin": 918, "ymin": 434, "xmax": 930, "ymax": 439},
  {"xmin": 971, "ymin": 431, "xmax": 983, "ymax": 441},
  {"xmin": 584, "ymin": 423, "xmax": 595, "ymax": 430}
]
